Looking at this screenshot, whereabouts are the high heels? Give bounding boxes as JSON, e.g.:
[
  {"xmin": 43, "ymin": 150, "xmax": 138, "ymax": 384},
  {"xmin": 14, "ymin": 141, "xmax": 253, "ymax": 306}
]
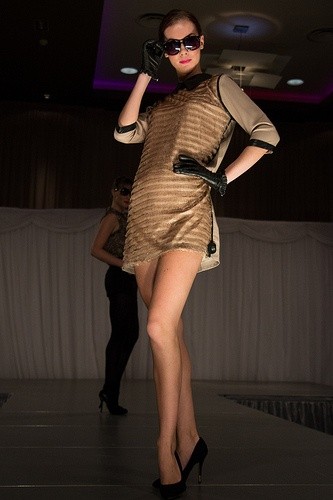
[
  {"xmin": 151, "ymin": 435, "xmax": 209, "ymax": 488},
  {"xmin": 98, "ymin": 390, "xmax": 129, "ymax": 415},
  {"xmin": 159, "ymin": 450, "xmax": 186, "ymax": 500}
]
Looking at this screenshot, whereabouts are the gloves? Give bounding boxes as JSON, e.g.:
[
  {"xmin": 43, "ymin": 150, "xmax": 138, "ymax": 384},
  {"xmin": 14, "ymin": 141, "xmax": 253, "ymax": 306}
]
[
  {"xmin": 138, "ymin": 36, "xmax": 167, "ymax": 79},
  {"xmin": 173, "ymin": 154, "xmax": 228, "ymax": 197}
]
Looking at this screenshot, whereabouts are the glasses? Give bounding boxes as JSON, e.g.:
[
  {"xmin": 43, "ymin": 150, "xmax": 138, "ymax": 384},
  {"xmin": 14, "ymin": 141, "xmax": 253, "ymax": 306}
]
[
  {"xmin": 118, "ymin": 188, "xmax": 130, "ymax": 196},
  {"xmin": 159, "ymin": 33, "xmax": 201, "ymax": 56}
]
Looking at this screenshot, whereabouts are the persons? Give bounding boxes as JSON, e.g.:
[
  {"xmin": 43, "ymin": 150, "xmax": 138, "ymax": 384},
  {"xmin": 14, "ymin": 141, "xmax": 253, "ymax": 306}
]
[
  {"xmin": 90, "ymin": 178, "xmax": 137, "ymax": 416},
  {"xmin": 114, "ymin": 10, "xmax": 280, "ymax": 500}
]
